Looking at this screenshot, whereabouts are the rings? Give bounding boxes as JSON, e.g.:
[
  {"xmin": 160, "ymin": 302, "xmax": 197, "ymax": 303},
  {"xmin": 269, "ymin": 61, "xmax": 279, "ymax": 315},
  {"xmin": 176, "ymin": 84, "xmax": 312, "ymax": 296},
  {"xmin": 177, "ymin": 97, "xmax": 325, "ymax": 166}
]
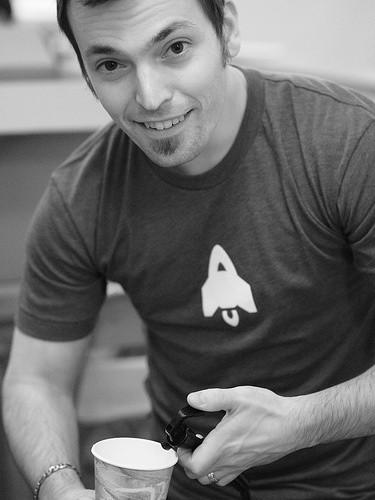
[{"xmin": 206, "ymin": 471, "xmax": 220, "ymax": 485}]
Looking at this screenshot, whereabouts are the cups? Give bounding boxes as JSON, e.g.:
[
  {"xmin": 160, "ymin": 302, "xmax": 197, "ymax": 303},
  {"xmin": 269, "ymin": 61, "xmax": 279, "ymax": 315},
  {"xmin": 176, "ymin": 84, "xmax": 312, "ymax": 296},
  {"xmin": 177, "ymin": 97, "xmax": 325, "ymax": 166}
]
[{"xmin": 90, "ymin": 437, "xmax": 178, "ymax": 500}]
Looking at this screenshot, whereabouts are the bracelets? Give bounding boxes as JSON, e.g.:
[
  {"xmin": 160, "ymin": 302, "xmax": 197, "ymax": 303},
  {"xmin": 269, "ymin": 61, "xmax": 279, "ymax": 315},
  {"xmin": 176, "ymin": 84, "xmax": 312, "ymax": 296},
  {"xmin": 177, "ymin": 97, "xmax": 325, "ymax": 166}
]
[{"xmin": 34, "ymin": 464, "xmax": 84, "ymax": 500}]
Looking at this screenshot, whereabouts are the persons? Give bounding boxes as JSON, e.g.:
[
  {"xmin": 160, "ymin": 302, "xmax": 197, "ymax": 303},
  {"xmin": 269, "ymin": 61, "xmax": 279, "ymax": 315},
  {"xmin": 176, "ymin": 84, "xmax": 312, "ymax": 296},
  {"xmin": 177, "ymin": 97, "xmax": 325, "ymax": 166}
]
[{"xmin": 0, "ymin": 0, "xmax": 375, "ymax": 500}]
